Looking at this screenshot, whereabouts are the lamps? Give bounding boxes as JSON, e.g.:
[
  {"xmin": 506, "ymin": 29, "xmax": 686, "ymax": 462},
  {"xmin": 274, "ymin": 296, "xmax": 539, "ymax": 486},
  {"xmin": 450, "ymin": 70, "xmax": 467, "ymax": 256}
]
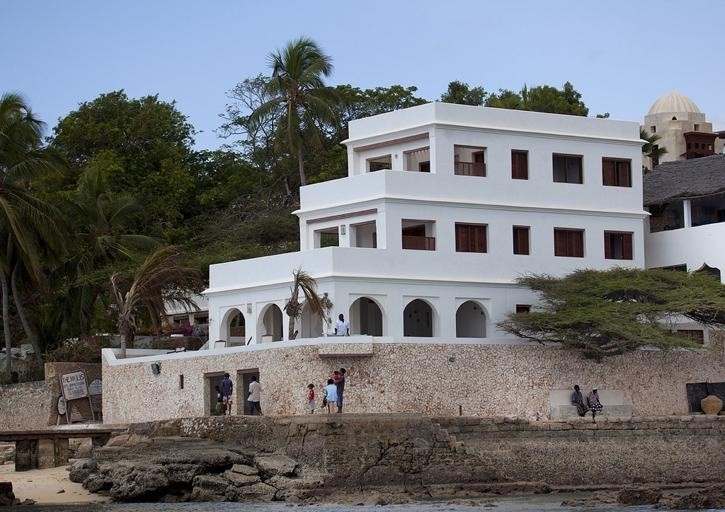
[{"xmin": 151, "ymin": 363, "xmax": 162, "ymax": 374}]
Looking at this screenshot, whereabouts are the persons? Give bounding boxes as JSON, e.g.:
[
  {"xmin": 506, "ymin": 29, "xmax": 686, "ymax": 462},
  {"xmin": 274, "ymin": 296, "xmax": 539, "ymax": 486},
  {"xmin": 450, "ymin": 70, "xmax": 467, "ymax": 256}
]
[
  {"xmin": 246, "ymin": 375, "xmax": 263, "ymax": 415},
  {"xmin": 320, "ymin": 379, "xmax": 338, "ymax": 407},
  {"xmin": 335, "ymin": 313, "xmax": 350, "ymax": 336},
  {"xmin": 324, "ymin": 316, "xmax": 336, "ymax": 335},
  {"xmin": 570, "ymin": 384, "xmax": 589, "ymax": 417},
  {"xmin": 307, "ymin": 383, "xmax": 316, "ymax": 414},
  {"xmin": 333, "ymin": 370, "xmax": 339, "ymax": 378},
  {"xmin": 219, "ymin": 373, "xmax": 233, "ymax": 415},
  {"xmin": 587, "ymin": 386, "xmax": 603, "ymax": 418},
  {"xmin": 331, "ymin": 367, "xmax": 347, "ymax": 414},
  {"xmin": 214, "ymin": 385, "xmax": 223, "ymax": 414}
]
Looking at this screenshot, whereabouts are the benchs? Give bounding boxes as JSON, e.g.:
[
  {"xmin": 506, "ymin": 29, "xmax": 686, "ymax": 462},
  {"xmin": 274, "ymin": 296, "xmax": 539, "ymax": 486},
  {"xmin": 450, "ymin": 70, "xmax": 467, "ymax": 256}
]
[{"xmin": 551, "ymin": 388, "xmax": 632, "ymax": 417}]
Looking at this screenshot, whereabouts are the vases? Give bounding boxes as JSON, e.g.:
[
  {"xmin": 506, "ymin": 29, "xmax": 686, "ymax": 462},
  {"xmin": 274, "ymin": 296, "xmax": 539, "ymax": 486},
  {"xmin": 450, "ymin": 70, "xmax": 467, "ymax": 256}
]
[{"xmin": 701, "ymin": 395, "xmax": 723, "ymax": 415}]
